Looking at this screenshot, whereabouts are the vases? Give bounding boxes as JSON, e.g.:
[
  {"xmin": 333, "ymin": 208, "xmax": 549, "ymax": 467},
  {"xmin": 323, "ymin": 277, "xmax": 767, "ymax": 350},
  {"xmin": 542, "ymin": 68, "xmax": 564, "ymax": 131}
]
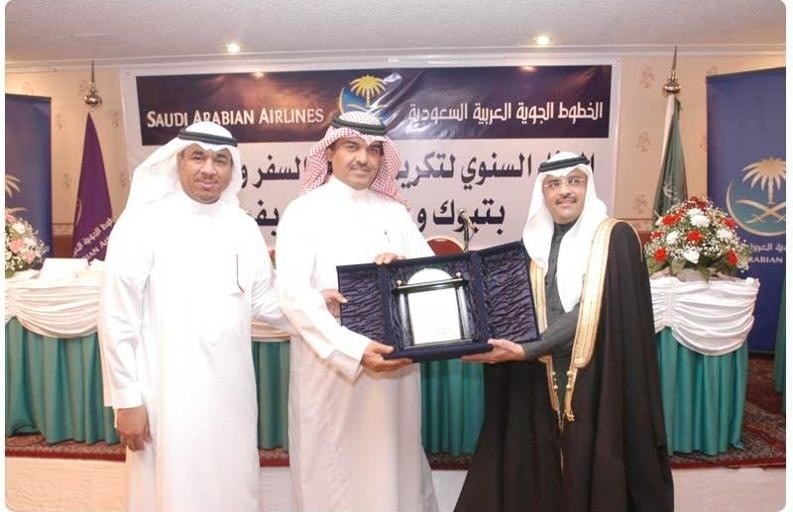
[
  {"xmin": 13, "ymin": 268, "xmax": 40, "ymax": 280},
  {"xmin": 676, "ymin": 269, "xmax": 705, "ymax": 282}
]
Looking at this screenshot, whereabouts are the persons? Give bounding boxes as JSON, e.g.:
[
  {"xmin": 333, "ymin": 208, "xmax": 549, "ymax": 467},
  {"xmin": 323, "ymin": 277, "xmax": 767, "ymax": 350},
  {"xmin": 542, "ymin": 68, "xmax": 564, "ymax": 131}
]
[
  {"xmin": 98, "ymin": 121, "xmax": 349, "ymax": 512},
  {"xmin": 453, "ymin": 151, "xmax": 675, "ymax": 512},
  {"xmin": 650, "ymin": 97, "xmax": 689, "ymax": 232},
  {"xmin": 273, "ymin": 109, "xmax": 439, "ymax": 512}
]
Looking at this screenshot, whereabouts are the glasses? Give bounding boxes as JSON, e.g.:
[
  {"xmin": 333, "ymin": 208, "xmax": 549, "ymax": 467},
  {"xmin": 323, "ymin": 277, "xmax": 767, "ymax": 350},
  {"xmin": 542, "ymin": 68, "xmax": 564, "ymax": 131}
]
[{"xmin": 544, "ymin": 179, "xmax": 586, "ymax": 188}]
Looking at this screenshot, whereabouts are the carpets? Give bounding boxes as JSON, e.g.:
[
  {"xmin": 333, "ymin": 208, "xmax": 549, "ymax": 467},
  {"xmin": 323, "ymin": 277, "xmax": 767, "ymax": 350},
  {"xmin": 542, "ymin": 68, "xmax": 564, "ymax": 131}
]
[{"xmin": 5, "ymin": 354, "xmax": 785, "ymax": 470}]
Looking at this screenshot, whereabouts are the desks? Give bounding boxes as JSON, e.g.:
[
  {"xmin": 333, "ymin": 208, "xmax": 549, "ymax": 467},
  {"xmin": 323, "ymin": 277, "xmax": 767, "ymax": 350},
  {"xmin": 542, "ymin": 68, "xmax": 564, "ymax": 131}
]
[{"xmin": 5, "ymin": 275, "xmax": 762, "ymax": 457}]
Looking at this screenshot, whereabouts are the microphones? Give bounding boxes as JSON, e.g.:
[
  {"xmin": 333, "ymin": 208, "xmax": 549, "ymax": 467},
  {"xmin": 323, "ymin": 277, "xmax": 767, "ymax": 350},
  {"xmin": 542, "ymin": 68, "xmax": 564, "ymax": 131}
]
[{"xmin": 458, "ymin": 207, "xmax": 478, "ymax": 233}]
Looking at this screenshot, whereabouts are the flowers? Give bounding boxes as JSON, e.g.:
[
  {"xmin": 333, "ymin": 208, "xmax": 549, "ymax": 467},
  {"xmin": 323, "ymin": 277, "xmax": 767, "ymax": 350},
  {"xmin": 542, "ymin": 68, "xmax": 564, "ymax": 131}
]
[
  {"xmin": 6, "ymin": 214, "xmax": 51, "ymax": 279},
  {"xmin": 642, "ymin": 195, "xmax": 752, "ymax": 284}
]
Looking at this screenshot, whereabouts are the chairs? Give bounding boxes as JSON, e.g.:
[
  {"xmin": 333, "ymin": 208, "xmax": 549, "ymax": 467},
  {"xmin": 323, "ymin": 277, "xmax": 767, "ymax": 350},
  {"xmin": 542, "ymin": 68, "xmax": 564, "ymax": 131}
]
[{"xmin": 426, "ymin": 236, "xmax": 465, "ymax": 256}]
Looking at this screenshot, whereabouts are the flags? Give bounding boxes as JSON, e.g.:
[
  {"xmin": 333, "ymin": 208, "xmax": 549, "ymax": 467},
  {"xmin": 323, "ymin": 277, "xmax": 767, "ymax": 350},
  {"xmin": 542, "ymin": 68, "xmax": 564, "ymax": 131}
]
[{"xmin": 70, "ymin": 113, "xmax": 114, "ymax": 260}]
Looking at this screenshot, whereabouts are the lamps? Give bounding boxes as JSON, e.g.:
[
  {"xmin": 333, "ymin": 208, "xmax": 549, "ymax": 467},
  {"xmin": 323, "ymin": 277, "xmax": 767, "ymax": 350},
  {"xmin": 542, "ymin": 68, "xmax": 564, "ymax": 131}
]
[
  {"xmin": 662, "ymin": 45, "xmax": 683, "ymax": 94},
  {"xmin": 82, "ymin": 59, "xmax": 101, "ymax": 106}
]
